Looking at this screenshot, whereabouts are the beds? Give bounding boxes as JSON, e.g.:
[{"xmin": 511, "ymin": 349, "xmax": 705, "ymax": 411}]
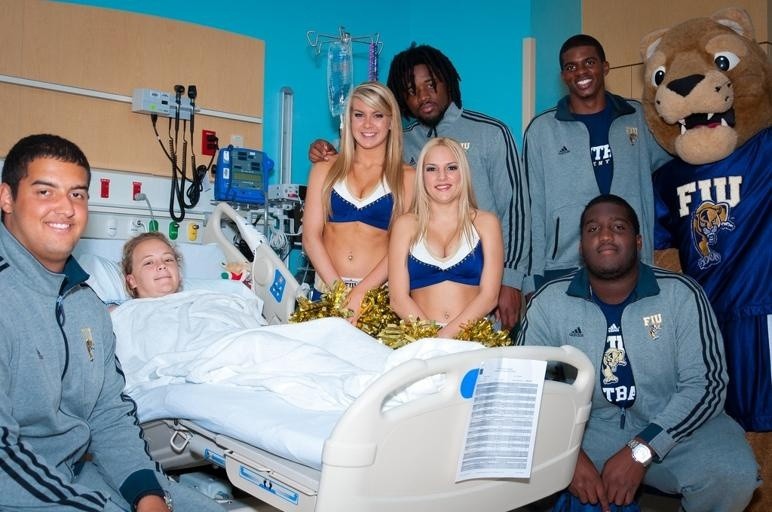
[{"xmin": 78, "ymin": 199, "xmax": 598, "ymax": 512}]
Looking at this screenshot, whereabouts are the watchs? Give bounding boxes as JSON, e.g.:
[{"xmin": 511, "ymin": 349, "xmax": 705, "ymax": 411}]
[{"xmin": 625, "ymin": 439, "xmax": 653, "ymax": 468}]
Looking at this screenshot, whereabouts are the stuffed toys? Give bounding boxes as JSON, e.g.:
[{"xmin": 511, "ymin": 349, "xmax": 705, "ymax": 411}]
[{"xmin": 640, "ymin": 5, "xmax": 772, "ymax": 492}]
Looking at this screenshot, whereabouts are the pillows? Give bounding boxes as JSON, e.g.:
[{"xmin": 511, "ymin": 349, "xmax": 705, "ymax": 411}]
[{"xmin": 79, "ymin": 251, "xmax": 265, "ymax": 318}]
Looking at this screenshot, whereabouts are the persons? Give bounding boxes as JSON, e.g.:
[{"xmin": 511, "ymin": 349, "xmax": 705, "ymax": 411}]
[
  {"xmin": 105, "ymin": 231, "xmax": 183, "ymax": 313},
  {"xmin": 515, "ymin": 195, "xmax": 759, "ymax": 512},
  {"xmin": 521, "ymin": 32, "xmax": 677, "ymax": 290},
  {"xmin": 302, "ymin": 40, "xmax": 530, "ymax": 338},
  {"xmin": 0, "ymin": 134, "xmax": 224, "ymax": 512}
]
